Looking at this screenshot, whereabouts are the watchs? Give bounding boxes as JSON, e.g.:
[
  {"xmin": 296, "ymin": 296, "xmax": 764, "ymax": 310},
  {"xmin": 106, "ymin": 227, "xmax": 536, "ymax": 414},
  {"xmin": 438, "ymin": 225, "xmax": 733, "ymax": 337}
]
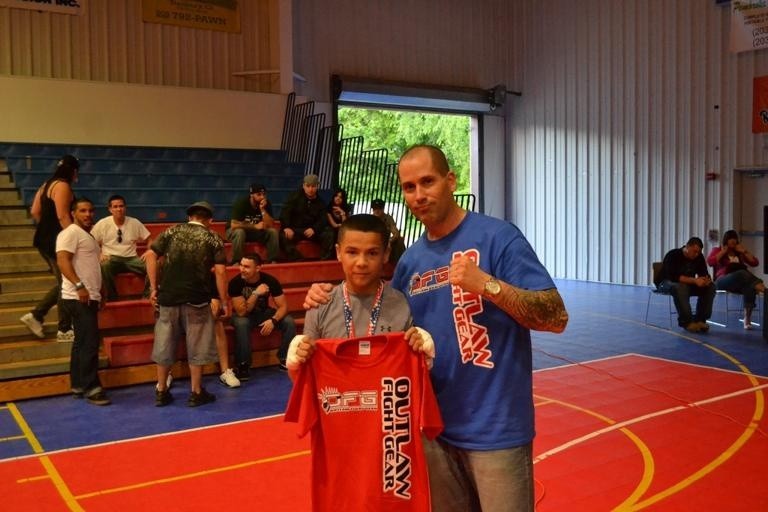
[
  {"xmin": 271, "ymin": 318, "xmax": 277, "ymax": 325},
  {"xmin": 480, "ymin": 274, "xmax": 503, "ymax": 303}
]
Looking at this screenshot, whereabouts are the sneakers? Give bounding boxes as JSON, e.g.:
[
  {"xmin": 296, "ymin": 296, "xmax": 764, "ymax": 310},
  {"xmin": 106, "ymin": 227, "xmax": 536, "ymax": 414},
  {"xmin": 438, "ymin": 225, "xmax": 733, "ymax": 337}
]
[
  {"xmin": 218, "ymin": 368, "xmax": 240, "ymax": 388},
  {"xmin": 279, "ymin": 363, "xmax": 288, "ymax": 372},
  {"xmin": 155, "ymin": 389, "xmax": 174, "ymax": 407},
  {"xmin": 56, "ymin": 329, "xmax": 74, "ymax": 342},
  {"xmin": 73, "ymin": 392, "xmax": 83, "ymax": 400},
  {"xmin": 155, "ymin": 371, "xmax": 173, "ymax": 392},
  {"xmin": 20, "ymin": 312, "xmax": 45, "ymax": 339},
  {"xmin": 86, "ymin": 392, "xmax": 112, "ymax": 406},
  {"xmin": 187, "ymin": 388, "xmax": 216, "ymax": 407},
  {"xmin": 236, "ymin": 364, "xmax": 248, "ymax": 381}
]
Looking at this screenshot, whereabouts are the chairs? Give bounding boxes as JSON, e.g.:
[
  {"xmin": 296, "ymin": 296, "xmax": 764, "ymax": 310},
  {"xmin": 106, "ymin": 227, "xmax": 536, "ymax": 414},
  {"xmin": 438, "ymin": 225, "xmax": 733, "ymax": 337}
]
[
  {"xmin": 644, "ymin": 262, "xmax": 678, "ymax": 325},
  {"xmin": 704, "ymin": 263, "xmax": 761, "ymax": 328}
]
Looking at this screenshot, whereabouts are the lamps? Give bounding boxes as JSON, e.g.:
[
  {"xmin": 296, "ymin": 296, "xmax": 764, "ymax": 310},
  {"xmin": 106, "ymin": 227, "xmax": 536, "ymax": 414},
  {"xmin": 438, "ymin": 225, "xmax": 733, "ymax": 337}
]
[{"xmin": 488, "ymin": 83, "xmax": 506, "ymax": 111}]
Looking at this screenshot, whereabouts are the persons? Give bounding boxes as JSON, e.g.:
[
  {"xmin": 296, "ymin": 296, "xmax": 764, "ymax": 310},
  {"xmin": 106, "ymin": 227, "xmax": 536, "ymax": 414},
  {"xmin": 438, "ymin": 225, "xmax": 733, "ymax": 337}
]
[
  {"xmin": 285, "ymin": 214, "xmax": 435, "ymax": 512},
  {"xmin": 658, "ymin": 235, "xmax": 715, "ymax": 332},
  {"xmin": 370, "ymin": 198, "xmax": 406, "ymax": 266},
  {"xmin": 226, "ymin": 183, "xmax": 279, "ymax": 266},
  {"xmin": 707, "ymin": 230, "xmax": 766, "ymax": 331},
  {"xmin": 19, "ymin": 156, "xmax": 81, "ymax": 343},
  {"xmin": 55, "ymin": 197, "xmax": 111, "ymax": 406},
  {"xmin": 90, "ymin": 195, "xmax": 152, "ymax": 301},
  {"xmin": 279, "ymin": 174, "xmax": 350, "ymax": 263},
  {"xmin": 227, "ymin": 252, "xmax": 296, "ymax": 381},
  {"xmin": 145, "ymin": 201, "xmax": 240, "ymax": 406},
  {"xmin": 302, "ymin": 145, "xmax": 569, "ymax": 512}
]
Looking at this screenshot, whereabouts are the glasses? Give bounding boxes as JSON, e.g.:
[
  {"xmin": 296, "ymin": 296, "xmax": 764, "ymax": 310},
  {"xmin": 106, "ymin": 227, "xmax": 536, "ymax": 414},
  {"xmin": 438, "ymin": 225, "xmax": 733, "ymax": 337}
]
[{"xmin": 116, "ymin": 229, "xmax": 122, "ymax": 243}]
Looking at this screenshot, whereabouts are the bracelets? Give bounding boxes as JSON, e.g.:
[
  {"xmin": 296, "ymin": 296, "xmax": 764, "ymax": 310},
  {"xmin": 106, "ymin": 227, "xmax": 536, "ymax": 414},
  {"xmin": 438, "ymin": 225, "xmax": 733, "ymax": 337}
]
[
  {"xmin": 252, "ymin": 291, "xmax": 260, "ymax": 297},
  {"xmin": 75, "ymin": 282, "xmax": 84, "ymax": 290}
]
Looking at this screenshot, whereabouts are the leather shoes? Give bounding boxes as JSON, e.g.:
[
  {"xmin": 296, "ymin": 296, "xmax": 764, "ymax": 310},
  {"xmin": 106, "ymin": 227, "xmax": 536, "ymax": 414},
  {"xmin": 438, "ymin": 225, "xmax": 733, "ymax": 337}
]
[
  {"xmin": 697, "ymin": 321, "xmax": 708, "ymax": 331},
  {"xmin": 686, "ymin": 322, "xmax": 699, "ymax": 332},
  {"xmin": 743, "ymin": 322, "xmax": 752, "ymax": 330}
]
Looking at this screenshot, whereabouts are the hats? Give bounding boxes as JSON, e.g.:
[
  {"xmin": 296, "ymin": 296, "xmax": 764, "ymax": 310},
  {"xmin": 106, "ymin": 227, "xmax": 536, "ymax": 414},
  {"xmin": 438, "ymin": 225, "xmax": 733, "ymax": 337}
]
[
  {"xmin": 304, "ymin": 174, "xmax": 321, "ymax": 186},
  {"xmin": 370, "ymin": 199, "xmax": 383, "ymax": 208},
  {"xmin": 248, "ymin": 183, "xmax": 266, "ymax": 194},
  {"xmin": 186, "ymin": 200, "xmax": 214, "ymax": 215}
]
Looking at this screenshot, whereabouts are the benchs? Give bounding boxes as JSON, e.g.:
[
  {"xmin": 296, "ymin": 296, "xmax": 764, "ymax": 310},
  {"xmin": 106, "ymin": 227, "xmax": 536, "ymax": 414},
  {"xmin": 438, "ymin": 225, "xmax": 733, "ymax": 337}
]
[{"xmin": 0, "ymin": 141, "xmax": 394, "ymax": 366}]
